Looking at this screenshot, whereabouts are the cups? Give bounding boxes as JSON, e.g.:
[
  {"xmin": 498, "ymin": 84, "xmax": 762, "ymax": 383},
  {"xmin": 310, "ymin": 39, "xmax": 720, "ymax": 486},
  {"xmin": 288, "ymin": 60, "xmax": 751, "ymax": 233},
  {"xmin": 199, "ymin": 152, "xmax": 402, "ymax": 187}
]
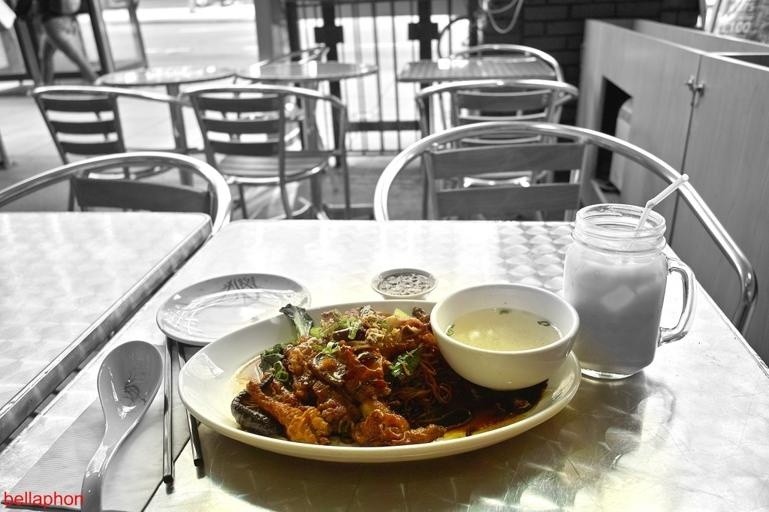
[{"xmin": 564, "ymin": 204, "xmax": 695, "ymax": 382}]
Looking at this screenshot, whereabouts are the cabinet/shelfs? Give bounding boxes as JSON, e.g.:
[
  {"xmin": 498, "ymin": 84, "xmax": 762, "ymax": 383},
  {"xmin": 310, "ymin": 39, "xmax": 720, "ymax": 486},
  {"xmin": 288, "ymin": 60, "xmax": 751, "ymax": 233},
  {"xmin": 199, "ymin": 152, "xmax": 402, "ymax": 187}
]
[{"xmin": 578, "ymin": 18, "xmax": 767, "ymax": 363}]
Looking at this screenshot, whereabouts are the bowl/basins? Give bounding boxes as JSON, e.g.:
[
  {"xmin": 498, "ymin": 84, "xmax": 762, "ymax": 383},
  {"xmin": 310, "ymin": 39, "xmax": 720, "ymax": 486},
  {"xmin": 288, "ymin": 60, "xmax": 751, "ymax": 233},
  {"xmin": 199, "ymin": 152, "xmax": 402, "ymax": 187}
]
[{"xmin": 429, "ymin": 282, "xmax": 581, "ymax": 392}]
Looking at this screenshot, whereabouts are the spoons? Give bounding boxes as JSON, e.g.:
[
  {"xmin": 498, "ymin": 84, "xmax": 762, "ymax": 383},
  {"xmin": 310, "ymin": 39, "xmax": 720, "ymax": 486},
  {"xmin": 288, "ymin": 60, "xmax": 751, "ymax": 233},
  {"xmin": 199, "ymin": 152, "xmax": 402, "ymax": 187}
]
[{"xmin": 80, "ymin": 340, "xmax": 164, "ymax": 512}]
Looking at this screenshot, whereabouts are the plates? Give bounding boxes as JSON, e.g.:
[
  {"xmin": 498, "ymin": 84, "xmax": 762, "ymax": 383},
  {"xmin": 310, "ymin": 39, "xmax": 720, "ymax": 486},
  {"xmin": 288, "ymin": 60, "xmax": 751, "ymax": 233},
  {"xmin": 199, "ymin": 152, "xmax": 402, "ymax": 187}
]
[
  {"xmin": 155, "ymin": 272, "xmax": 310, "ymax": 347},
  {"xmin": 373, "ymin": 268, "xmax": 439, "ymax": 300},
  {"xmin": 176, "ymin": 302, "xmax": 581, "ymax": 464}
]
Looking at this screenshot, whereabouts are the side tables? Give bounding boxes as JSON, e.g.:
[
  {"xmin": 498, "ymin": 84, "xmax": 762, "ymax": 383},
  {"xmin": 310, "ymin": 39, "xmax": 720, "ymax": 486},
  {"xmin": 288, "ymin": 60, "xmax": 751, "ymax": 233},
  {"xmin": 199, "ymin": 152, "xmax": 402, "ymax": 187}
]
[{"xmin": 0, "ymin": 211, "xmax": 769, "ymax": 512}]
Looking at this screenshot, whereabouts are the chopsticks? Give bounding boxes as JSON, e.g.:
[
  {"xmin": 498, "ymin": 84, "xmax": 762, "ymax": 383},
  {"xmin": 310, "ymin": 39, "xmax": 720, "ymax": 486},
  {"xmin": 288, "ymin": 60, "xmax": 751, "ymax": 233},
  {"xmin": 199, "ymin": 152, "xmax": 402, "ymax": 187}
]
[{"xmin": 162, "ymin": 335, "xmax": 203, "ymax": 482}]
